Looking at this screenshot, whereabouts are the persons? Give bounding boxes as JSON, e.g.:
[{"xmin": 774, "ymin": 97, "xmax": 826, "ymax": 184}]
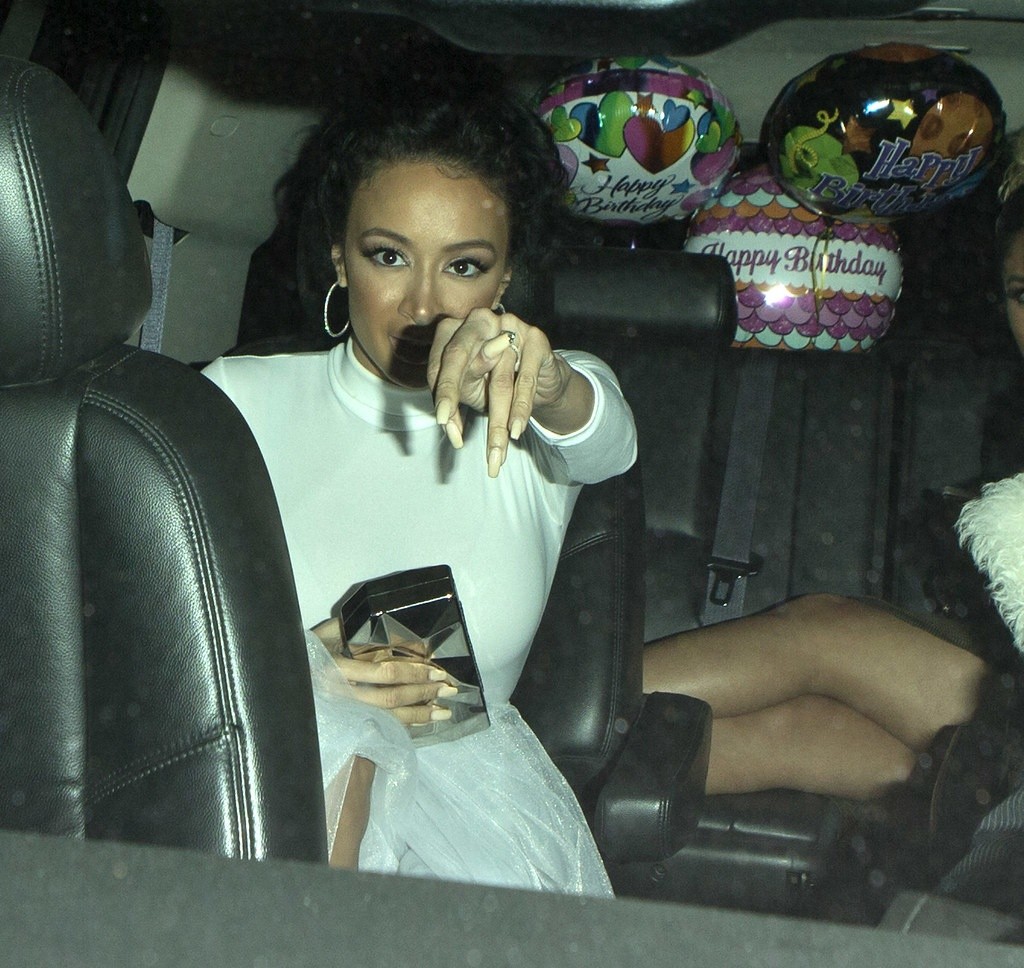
[
  {"xmin": 193, "ymin": 96, "xmax": 640, "ymax": 898},
  {"xmin": 639, "ymin": 192, "xmax": 1023, "ymax": 885}
]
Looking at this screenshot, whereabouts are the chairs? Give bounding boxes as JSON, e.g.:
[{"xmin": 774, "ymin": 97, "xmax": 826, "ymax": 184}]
[{"xmin": 0, "ymin": 60, "xmax": 1024, "ymax": 954}]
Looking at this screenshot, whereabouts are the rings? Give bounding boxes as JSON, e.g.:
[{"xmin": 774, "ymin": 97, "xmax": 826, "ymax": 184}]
[{"xmin": 510, "ymin": 343, "xmax": 521, "ymax": 370}]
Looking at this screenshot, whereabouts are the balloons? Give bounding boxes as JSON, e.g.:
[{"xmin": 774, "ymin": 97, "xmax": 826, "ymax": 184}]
[{"xmin": 534, "ymin": 39, "xmax": 1010, "ymax": 357}]
